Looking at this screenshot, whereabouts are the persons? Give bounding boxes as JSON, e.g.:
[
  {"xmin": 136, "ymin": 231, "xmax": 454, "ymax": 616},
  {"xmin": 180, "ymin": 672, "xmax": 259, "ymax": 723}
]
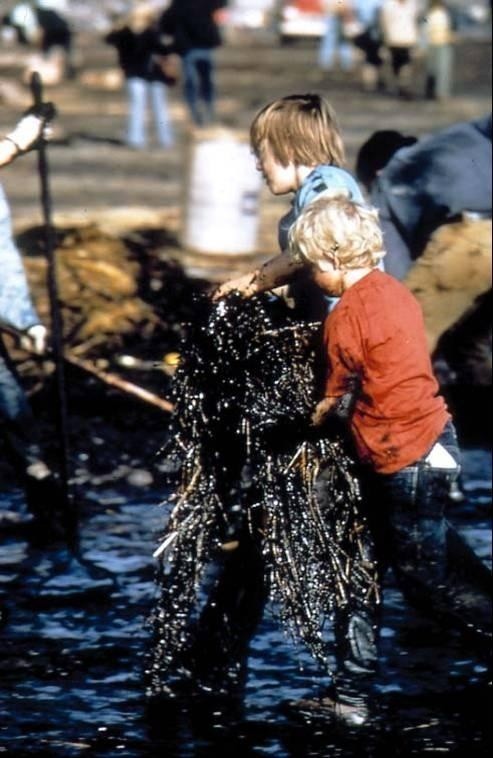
[
  {"xmin": 350, "ymin": 113, "xmax": 493, "ymax": 363},
  {"xmin": 288, "ymin": 197, "xmax": 491, "ymax": 723},
  {"xmin": 0, "ymin": 4, "xmax": 72, "ymax": 85},
  {"xmin": 319, "ymin": 1, "xmax": 457, "ymax": 102},
  {"xmin": 106, "ymin": 0, "xmax": 229, "ymax": 148},
  {"xmin": 0, "ymin": 105, "xmax": 56, "ymax": 558},
  {"xmin": 209, "ymin": 95, "xmax": 463, "ymax": 517}
]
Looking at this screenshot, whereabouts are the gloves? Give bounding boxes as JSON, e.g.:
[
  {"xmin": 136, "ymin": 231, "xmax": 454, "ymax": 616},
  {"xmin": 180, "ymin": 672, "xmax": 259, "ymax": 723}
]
[
  {"xmin": 19, "ymin": 325, "xmax": 57, "ymax": 376},
  {"xmin": 5, "ymin": 101, "xmax": 57, "ymax": 151}
]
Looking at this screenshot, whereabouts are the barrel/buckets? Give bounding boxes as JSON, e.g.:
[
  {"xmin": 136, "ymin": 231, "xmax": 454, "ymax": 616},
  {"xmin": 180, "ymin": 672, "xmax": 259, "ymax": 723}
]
[{"xmin": 182, "ymin": 134, "xmax": 260, "ymax": 255}]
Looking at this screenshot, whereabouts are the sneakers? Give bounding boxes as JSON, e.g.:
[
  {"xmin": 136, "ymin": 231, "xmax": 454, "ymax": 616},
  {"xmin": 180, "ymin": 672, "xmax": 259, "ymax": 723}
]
[
  {"xmin": 284, "ymin": 691, "xmax": 367, "ymax": 727},
  {"xmin": 22, "ymin": 546, "xmax": 114, "ymax": 594}
]
